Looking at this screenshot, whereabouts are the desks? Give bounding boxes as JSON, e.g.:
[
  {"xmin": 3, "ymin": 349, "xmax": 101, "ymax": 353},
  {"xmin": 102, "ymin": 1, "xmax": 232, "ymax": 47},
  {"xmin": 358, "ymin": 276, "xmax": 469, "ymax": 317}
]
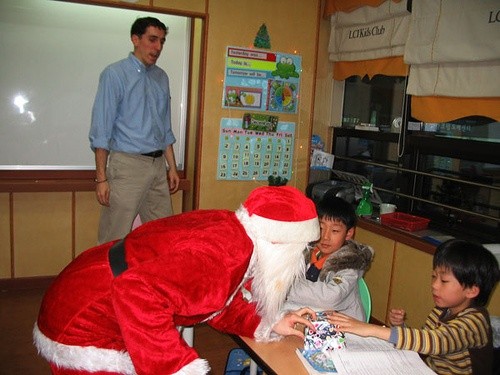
[{"xmin": 233, "ymin": 336, "xmax": 309, "ymax": 375}]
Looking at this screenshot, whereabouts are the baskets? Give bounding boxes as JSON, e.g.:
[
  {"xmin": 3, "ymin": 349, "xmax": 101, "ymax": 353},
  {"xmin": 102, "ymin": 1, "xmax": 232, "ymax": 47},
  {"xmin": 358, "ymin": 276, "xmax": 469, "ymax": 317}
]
[{"xmin": 380, "ymin": 211, "xmax": 431, "ymax": 232}]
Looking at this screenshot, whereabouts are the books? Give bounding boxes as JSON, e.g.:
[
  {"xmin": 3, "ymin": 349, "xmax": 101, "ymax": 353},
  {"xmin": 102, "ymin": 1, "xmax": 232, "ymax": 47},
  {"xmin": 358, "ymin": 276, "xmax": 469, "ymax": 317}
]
[{"xmin": 296, "ymin": 333, "xmax": 435, "ymax": 374}]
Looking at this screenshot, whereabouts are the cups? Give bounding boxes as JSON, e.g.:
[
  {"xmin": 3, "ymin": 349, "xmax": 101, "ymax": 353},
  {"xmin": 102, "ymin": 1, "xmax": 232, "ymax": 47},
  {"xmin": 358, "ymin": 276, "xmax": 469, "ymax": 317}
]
[{"xmin": 379, "ymin": 203, "xmax": 396, "ymax": 214}]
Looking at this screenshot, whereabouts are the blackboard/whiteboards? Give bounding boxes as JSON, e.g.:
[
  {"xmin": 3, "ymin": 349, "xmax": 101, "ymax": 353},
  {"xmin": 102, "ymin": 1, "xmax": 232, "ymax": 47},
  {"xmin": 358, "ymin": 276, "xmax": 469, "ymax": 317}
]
[{"xmin": 0, "ymin": 0, "xmax": 195, "ymax": 193}]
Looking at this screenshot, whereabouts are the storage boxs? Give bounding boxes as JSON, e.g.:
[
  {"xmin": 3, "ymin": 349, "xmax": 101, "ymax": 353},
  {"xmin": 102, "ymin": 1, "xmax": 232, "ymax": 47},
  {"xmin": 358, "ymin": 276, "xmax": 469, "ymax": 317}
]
[{"xmin": 380, "ymin": 212, "xmax": 430, "ymax": 233}]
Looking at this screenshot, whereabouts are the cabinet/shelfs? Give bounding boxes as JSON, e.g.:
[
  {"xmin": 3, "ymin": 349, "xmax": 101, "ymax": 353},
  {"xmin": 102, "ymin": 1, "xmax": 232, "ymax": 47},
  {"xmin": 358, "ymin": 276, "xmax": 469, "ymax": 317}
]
[{"xmin": 353, "ymin": 218, "xmax": 500, "ymax": 329}]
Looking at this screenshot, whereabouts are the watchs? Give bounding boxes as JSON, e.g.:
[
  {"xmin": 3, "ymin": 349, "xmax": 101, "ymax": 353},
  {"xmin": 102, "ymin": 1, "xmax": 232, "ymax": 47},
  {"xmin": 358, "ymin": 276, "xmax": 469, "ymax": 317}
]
[{"xmin": 94, "ymin": 177, "xmax": 108, "ymax": 184}]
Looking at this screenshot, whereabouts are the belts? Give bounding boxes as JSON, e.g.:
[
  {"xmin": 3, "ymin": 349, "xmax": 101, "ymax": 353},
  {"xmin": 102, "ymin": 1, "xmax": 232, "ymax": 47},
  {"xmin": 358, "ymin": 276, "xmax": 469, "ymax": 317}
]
[{"xmin": 142, "ymin": 149, "xmax": 163, "ymax": 157}]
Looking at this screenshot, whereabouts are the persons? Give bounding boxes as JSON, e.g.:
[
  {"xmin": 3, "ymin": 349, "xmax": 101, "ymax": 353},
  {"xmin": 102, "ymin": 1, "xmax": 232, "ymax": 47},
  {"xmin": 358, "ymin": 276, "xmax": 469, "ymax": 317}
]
[
  {"xmin": 327, "ymin": 239, "xmax": 500, "ymax": 375},
  {"xmin": 89, "ymin": 17, "xmax": 181, "ymax": 244},
  {"xmin": 34, "ymin": 184, "xmax": 317, "ymax": 375},
  {"xmin": 285, "ymin": 196, "xmax": 375, "ymax": 324}
]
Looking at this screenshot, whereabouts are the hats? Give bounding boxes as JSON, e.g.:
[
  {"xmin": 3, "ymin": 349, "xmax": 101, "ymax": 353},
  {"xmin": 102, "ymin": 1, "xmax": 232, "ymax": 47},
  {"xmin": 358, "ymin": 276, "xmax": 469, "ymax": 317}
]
[{"xmin": 238, "ymin": 176, "xmax": 321, "ymax": 244}]
